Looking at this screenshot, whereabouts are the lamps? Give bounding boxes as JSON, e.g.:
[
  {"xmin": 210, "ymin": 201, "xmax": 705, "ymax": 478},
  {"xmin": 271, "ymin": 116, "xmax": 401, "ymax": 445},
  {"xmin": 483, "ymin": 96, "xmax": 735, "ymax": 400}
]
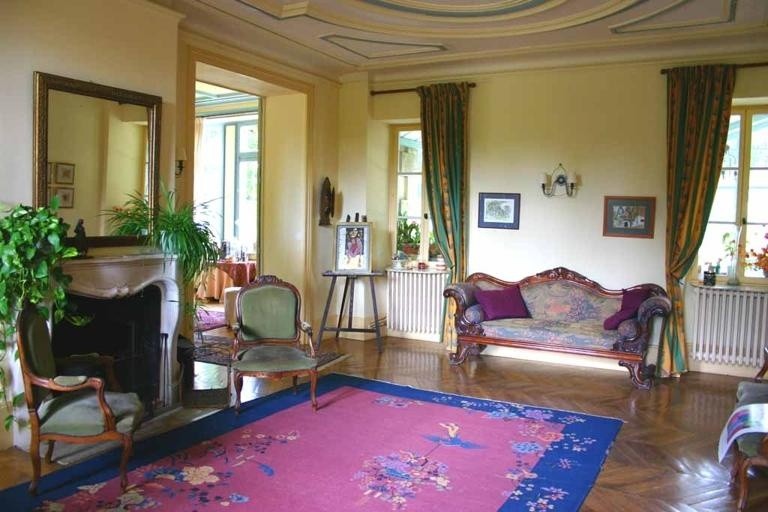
[{"xmin": 537, "ymin": 160, "xmax": 580, "ymax": 198}]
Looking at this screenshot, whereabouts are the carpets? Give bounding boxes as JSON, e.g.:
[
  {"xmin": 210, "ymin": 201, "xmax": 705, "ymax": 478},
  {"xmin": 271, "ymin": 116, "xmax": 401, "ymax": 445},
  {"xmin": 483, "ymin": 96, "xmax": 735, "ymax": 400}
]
[{"xmin": 2, "ymin": 369, "xmax": 626, "ymax": 512}]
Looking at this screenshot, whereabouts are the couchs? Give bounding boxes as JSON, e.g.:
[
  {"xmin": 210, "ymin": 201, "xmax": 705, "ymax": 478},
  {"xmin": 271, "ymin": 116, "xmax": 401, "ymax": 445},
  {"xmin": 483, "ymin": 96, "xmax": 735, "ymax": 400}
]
[{"xmin": 441, "ymin": 266, "xmax": 674, "ymax": 391}]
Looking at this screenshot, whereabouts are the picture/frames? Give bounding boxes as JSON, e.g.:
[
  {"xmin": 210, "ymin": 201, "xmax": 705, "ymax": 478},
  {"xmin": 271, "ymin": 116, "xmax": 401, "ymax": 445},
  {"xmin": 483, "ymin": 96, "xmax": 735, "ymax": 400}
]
[
  {"xmin": 334, "ymin": 221, "xmax": 371, "ymax": 271},
  {"xmin": 602, "ymin": 195, "xmax": 657, "ymax": 239},
  {"xmin": 477, "ymin": 190, "xmax": 521, "ymax": 231}
]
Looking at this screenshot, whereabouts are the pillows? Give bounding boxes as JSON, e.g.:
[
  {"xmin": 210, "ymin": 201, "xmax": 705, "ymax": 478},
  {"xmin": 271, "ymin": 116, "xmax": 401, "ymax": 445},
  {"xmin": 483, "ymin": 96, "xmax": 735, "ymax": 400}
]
[
  {"xmin": 470, "ymin": 281, "xmax": 532, "ymax": 323},
  {"xmin": 600, "ymin": 286, "xmax": 652, "ymax": 335}
]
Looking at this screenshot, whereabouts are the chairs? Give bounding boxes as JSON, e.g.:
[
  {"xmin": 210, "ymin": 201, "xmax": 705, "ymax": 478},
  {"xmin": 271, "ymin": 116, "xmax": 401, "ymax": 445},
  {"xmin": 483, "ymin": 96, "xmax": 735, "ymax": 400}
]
[
  {"xmin": 14, "ymin": 301, "xmax": 148, "ymax": 490},
  {"xmin": 226, "ymin": 272, "xmax": 319, "ymax": 417},
  {"xmin": 731, "ymin": 370, "xmax": 768, "ymax": 511}
]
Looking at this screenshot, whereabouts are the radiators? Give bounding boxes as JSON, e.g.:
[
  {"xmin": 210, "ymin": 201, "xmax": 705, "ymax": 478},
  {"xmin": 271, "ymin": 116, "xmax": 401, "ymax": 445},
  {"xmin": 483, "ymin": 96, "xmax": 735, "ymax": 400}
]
[
  {"xmin": 384, "ymin": 267, "xmax": 450, "ymax": 343},
  {"xmin": 685, "ymin": 282, "xmax": 768, "ymax": 377}
]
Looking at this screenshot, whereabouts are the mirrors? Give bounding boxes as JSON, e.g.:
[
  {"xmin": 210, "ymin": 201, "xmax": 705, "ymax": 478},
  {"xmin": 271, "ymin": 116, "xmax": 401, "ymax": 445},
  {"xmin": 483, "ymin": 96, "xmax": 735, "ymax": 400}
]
[{"xmin": 32, "ymin": 70, "xmax": 163, "ymax": 249}]
